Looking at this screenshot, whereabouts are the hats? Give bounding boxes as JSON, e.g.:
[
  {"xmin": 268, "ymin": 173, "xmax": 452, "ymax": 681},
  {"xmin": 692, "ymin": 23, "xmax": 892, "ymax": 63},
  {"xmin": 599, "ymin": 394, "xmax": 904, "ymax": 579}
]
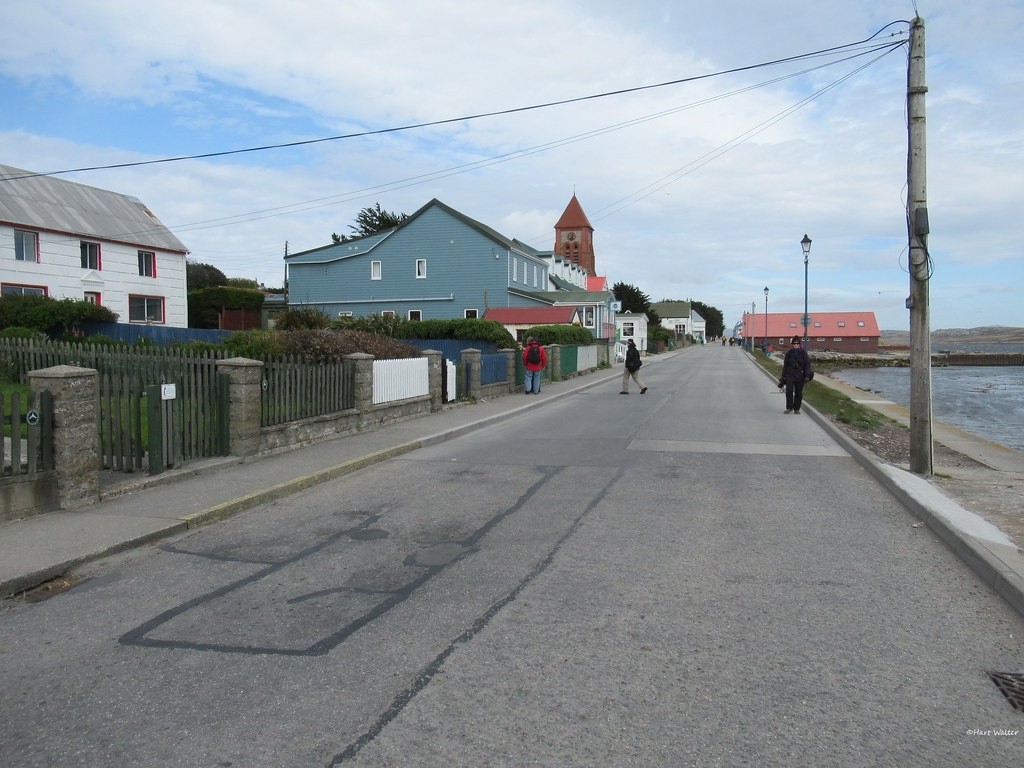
[
  {"xmin": 628, "ymin": 339, "xmax": 636, "ymax": 346},
  {"xmin": 792, "ymin": 335, "xmax": 800, "ymax": 343}
]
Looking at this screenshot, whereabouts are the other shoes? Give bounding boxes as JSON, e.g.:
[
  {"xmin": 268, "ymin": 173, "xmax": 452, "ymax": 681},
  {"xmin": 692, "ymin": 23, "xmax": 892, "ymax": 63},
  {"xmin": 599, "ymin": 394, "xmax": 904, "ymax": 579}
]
[
  {"xmin": 640, "ymin": 388, "xmax": 647, "ymax": 394},
  {"xmin": 525, "ymin": 391, "xmax": 530, "ymax": 394},
  {"xmin": 794, "ymin": 409, "xmax": 800, "ymax": 414},
  {"xmin": 620, "ymin": 392, "xmax": 629, "ymax": 394},
  {"xmin": 784, "ymin": 409, "xmax": 792, "ymax": 414}
]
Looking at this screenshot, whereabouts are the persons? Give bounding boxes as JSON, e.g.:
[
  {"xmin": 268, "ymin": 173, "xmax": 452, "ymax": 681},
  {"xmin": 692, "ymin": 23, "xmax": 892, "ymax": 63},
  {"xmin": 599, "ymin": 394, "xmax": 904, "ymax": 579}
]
[
  {"xmin": 721, "ymin": 335, "xmax": 776, "ymax": 358},
  {"xmin": 777, "ymin": 335, "xmax": 814, "ymax": 414},
  {"xmin": 522, "ymin": 338, "xmax": 547, "ymax": 394},
  {"xmin": 620, "ymin": 339, "xmax": 648, "ymax": 394}
]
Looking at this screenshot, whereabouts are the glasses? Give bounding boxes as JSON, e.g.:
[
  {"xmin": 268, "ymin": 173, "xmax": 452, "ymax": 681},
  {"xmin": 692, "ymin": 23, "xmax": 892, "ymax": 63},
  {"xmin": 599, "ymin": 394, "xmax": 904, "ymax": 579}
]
[{"xmin": 793, "ymin": 343, "xmax": 799, "ymax": 345}]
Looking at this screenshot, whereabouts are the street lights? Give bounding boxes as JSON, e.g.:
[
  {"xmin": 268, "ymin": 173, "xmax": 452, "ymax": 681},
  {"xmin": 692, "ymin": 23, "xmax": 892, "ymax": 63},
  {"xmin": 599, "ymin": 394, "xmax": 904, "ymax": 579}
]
[
  {"xmin": 800, "ymin": 234, "xmax": 812, "ymax": 352},
  {"xmin": 764, "ymin": 286, "xmax": 769, "ymax": 357},
  {"xmin": 752, "ymin": 302, "xmax": 756, "ymax": 353}
]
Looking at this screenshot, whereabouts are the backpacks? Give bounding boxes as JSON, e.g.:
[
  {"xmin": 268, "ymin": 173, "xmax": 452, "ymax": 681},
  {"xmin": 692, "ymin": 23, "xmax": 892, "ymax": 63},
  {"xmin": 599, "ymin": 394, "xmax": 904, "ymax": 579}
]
[
  {"xmin": 527, "ymin": 344, "xmax": 542, "ymax": 364},
  {"xmin": 628, "ymin": 360, "xmax": 642, "ymax": 372}
]
